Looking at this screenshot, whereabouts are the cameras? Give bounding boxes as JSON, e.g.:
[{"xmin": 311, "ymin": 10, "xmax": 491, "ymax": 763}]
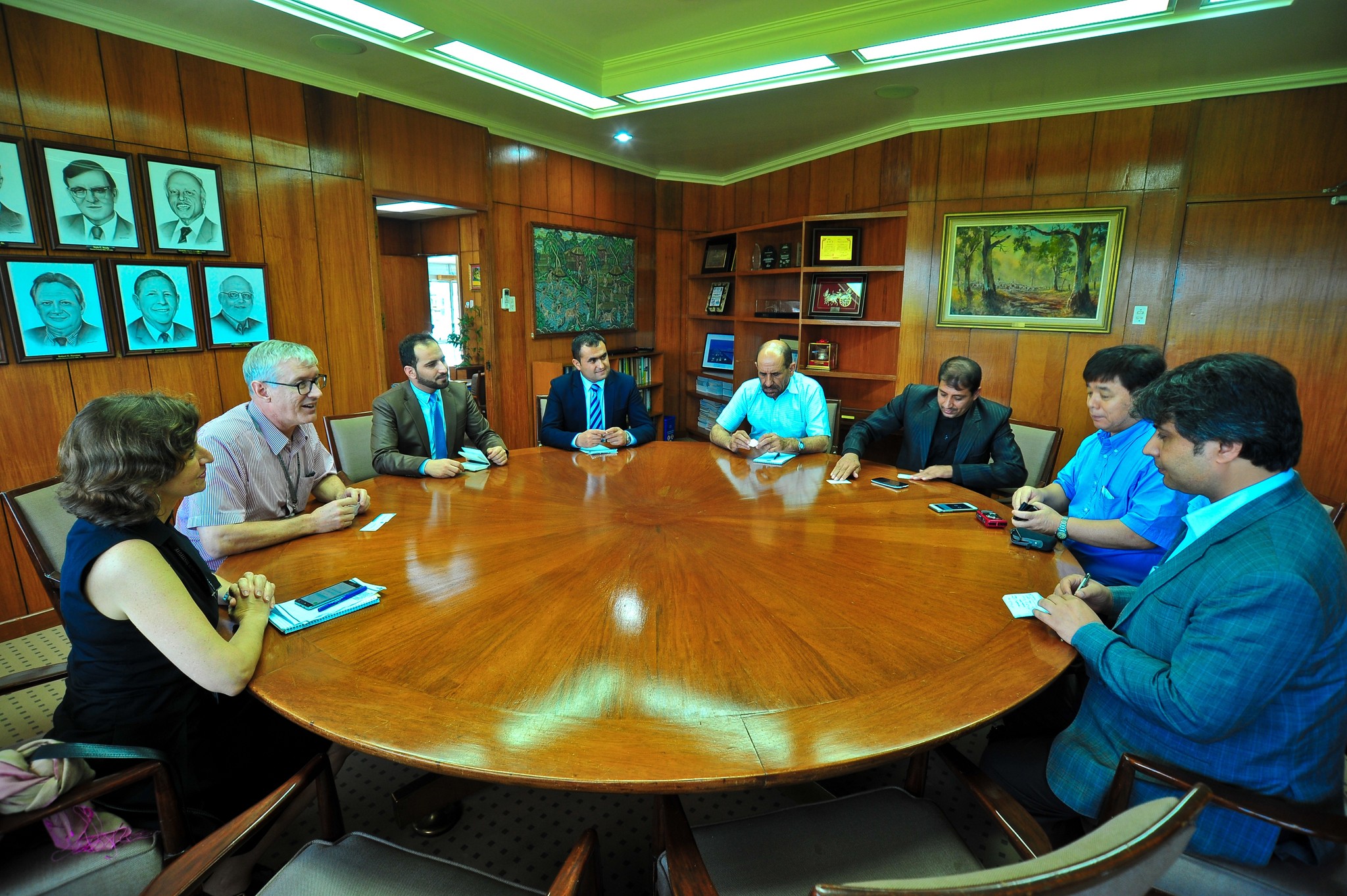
[{"xmin": 976, "ymin": 509, "xmax": 1008, "ymax": 528}]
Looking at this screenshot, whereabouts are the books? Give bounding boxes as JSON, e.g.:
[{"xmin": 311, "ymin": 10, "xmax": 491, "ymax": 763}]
[
  {"xmin": 694, "ymin": 375, "xmax": 734, "ymax": 435},
  {"xmin": 752, "ymin": 450, "xmax": 796, "ymax": 465},
  {"xmin": 580, "ymin": 443, "xmax": 618, "ymax": 455},
  {"xmin": 458, "ymin": 447, "xmax": 491, "ymax": 472},
  {"xmin": 1003, "ymin": 591, "xmax": 1048, "ymax": 620},
  {"xmin": 268, "ymin": 577, "xmax": 386, "ymax": 635},
  {"xmin": 461, "ymin": 470, "xmax": 491, "ymax": 490},
  {"xmin": 563, "ymin": 350, "xmax": 653, "ymax": 418}
]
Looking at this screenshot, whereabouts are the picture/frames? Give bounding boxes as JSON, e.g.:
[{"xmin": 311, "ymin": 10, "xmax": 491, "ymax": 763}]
[
  {"xmin": 705, "ymin": 282, "xmax": 729, "ymax": 313},
  {"xmin": 808, "ymin": 273, "xmax": 867, "ymax": 318},
  {"xmin": 779, "ymin": 334, "xmax": 799, "ymax": 373},
  {"xmin": 32, "ymin": 138, "xmax": 146, "ymax": 254},
  {"xmin": 1, "ymin": 135, "xmax": 43, "ymax": 251},
  {"xmin": 139, "ymin": 152, "xmax": 230, "ymax": 255},
  {"xmin": 0, "ymin": 253, "xmax": 116, "ymax": 362},
  {"xmin": 701, "ymin": 240, "xmax": 734, "ymax": 273},
  {"xmin": 197, "ymin": 260, "xmax": 274, "ymax": 350},
  {"xmin": 701, "ymin": 332, "xmax": 734, "ymax": 370},
  {"xmin": 528, "ymin": 220, "xmax": 638, "ymax": 340},
  {"xmin": 106, "ymin": 257, "xmax": 204, "ymax": 357},
  {"xmin": 935, "ymin": 206, "xmax": 1127, "ymax": 333},
  {"xmin": 813, "ymin": 227, "xmax": 859, "ymax": 267},
  {"xmin": 468, "ymin": 263, "xmax": 481, "ymax": 291}
]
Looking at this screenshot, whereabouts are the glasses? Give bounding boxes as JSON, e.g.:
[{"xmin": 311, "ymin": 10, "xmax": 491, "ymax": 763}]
[
  {"xmin": 67, "ymin": 185, "xmax": 110, "ymax": 198},
  {"xmin": 262, "ymin": 374, "xmax": 327, "ymax": 395},
  {"xmin": 219, "ymin": 291, "xmax": 253, "ymax": 299}
]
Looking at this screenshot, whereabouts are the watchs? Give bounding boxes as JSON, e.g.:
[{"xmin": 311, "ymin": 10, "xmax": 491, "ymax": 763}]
[
  {"xmin": 626, "ymin": 450, "xmax": 631, "ymax": 464},
  {"xmin": 793, "ymin": 438, "xmax": 805, "ymax": 454},
  {"xmin": 1054, "ymin": 515, "xmax": 1069, "ymax": 540},
  {"xmin": 625, "ymin": 431, "xmax": 631, "ymax": 445}
]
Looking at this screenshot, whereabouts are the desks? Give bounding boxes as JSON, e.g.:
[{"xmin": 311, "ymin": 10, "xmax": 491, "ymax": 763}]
[{"xmin": 213, "ymin": 441, "xmax": 1084, "ymax": 796}]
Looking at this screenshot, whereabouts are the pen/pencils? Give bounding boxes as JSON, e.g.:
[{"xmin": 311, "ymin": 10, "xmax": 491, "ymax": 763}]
[
  {"xmin": 1074, "ymin": 573, "xmax": 1092, "ymax": 598},
  {"xmin": 467, "ymin": 459, "xmax": 490, "ymax": 466},
  {"xmin": 774, "ymin": 452, "xmax": 780, "ymax": 460},
  {"xmin": 318, "ymin": 586, "xmax": 367, "ymax": 611}
]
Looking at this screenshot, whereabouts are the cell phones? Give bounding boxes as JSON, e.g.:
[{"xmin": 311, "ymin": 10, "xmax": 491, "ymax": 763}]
[
  {"xmin": 928, "ymin": 503, "xmax": 979, "ymax": 512},
  {"xmin": 294, "ymin": 578, "xmax": 361, "ymax": 611},
  {"xmin": 871, "ymin": 477, "xmax": 910, "ymax": 489}
]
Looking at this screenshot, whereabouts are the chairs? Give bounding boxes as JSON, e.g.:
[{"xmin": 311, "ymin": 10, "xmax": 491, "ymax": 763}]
[
  {"xmin": 323, "ymin": 411, "xmax": 382, "ymax": 482},
  {"xmin": 1099, "ymin": 753, "xmax": 1347, "ymax": 896},
  {"xmin": 0, "ymin": 662, "xmax": 182, "ymax": 896},
  {"xmin": 654, "ymin": 746, "xmax": 1213, "ymax": 896},
  {"xmin": 1, "ymin": 473, "xmax": 79, "ymax": 626},
  {"xmin": 826, "ymin": 399, "xmax": 841, "ymax": 455},
  {"xmin": 988, "ymin": 418, "xmax": 1064, "ymax": 509},
  {"xmin": 142, "ymin": 754, "xmax": 608, "ymax": 896}
]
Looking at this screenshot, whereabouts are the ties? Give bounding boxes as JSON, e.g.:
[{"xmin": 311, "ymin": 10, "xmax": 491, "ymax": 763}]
[
  {"xmin": 161, "ymin": 333, "xmax": 169, "ymax": 343},
  {"xmin": 590, "ymin": 384, "xmax": 604, "ymax": 443},
  {"xmin": 428, "ymin": 393, "xmax": 448, "ymax": 459},
  {"xmin": 91, "ymin": 226, "xmax": 103, "ymax": 240},
  {"xmin": 55, "ymin": 337, "xmax": 67, "ymax": 346},
  {"xmin": 178, "ymin": 227, "xmax": 192, "ymax": 243}
]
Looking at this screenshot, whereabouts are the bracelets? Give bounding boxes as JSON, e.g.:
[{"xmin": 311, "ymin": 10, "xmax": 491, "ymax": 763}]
[{"xmin": 223, "ymin": 590, "xmax": 230, "ymax": 603}]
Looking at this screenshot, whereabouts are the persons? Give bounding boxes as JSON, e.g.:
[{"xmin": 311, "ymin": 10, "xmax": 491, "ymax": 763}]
[
  {"xmin": 43, "ymin": 392, "xmax": 353, "ymax": 896},
  {"xmin": 157, "ymin": 168, "xmax": 218, "ymax": 248},
  {"xmin": 0, "ymin": 166, "xmax": 28, "ymax": 232},
  {"xmin": 369, "ymin": 333, "xmax": 509, "ymax": 479},
  {"xmin": 570, "ymin": 447, "xmax": 638, "ymax": 502},
  {"xmin": 708, "ymin": 441, "xmax": 828, "ymax": 544},
  {"xmin": 59, "ymin": 159, "xmax": 134, "ymax": 245},
  {"xmin": 1011, "ymin": 345, "xmax": 1199, "ymax": 588},
  {"xmin": 24, "ymin": 272, "xmax": 106, "ymax": 353},
  {"xmin": 981, "ymin": 353, "xmax": 1346, "ymax": 874},
  {"xmin": 709, "ymin": 340, "xmax": 832, "ymax": 454},
  {"xmin": 539, "ymin": 332, "xmax": 656, "ymax": 450},
  {"xmin": 830, "ymin": 357, "xmax": 985, "ymax": 480},
  {"xmin": 401, "ymin": 465, "xmax": 509, "ymax": 567},
  {"xmin": 210, "ymin": 275, "xmax": 262, "ymax": 335},
  {"xmin": 174, "ymin": 340, "xmax": 370, "ymax": 572},
  {"xmin": 125, "ymin": 269, "xmax": 194, "ymax": 345}
]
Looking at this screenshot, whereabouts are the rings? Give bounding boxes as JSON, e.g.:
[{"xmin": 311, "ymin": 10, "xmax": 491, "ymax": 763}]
[{"xmin": 457, "ymin": 468, "xmax": 460, "ymax": 473}]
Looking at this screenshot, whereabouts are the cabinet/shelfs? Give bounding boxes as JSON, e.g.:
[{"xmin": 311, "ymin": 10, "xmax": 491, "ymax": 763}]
[
  {"xmin": 532, "ymin": 351, "xmax": 664, "ymax": 447},
  {"xmin": 681, "ymin": 210, "xmax": 909, "ymax": 440}
]
